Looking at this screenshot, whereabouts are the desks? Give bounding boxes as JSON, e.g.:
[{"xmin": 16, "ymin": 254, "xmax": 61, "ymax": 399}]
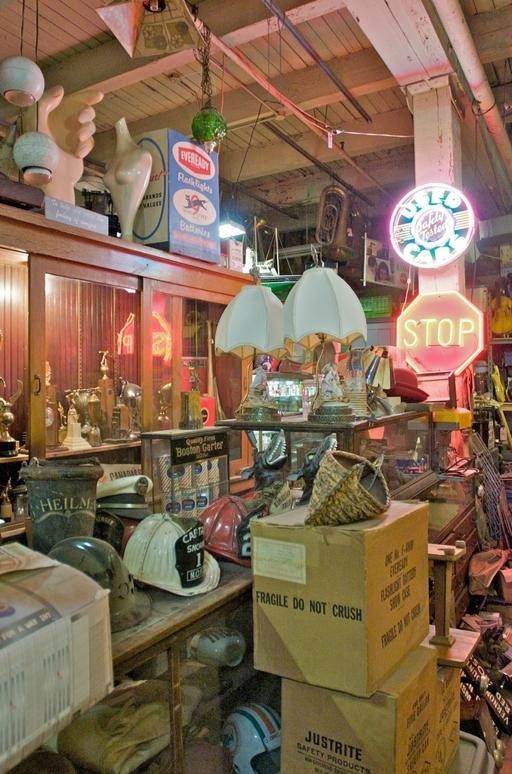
[{"xmin": 112, "ymin": 560, "xmax": 254, "ymax": 774}]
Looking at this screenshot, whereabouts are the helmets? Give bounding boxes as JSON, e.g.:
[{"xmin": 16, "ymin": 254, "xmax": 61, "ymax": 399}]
[
  {"xmin": 221, "ymin": 700, "xmax": 281, "ymax": 774},
  {"xmin": 45, "ymin": 532, "xmax": 154, "ymax": 636},
  {"xmin": 196, "ymin": 492, "xmax": 251, "ymax": 566},
  {"xmin": 120, "ymin": 510, "xmax": 223, "ymax": 597}
]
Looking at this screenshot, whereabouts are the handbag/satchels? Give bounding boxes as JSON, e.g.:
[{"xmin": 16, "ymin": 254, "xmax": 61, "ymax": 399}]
[{"xmin": 304, "ymin": 447, "xmax": 392, "ymax": 528}]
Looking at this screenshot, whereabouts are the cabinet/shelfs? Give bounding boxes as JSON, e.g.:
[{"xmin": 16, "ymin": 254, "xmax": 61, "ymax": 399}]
[
  {"xmin": 399, "ymin": 473, "xmax": 480, "ymax": 629},
  {"xmin": 1, "ymin": 204, "xmax": 261, "ymax": 537},
  {"xmin": 485, "ymin": 289, "xmax": 512, "ymax": 487}
]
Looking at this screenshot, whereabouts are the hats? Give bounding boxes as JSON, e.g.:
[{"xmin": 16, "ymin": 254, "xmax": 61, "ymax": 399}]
[
  {"xmin": 97, "ymin": 473, "xmax": 155, "ymax": 520},
  {"xmin": 383, "ymin": 366, "xmax": 430, "ymax": 403}
]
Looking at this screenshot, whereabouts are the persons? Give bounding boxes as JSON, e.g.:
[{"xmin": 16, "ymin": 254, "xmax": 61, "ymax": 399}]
[
  {"xmin": 21, "ymin": 85, "xmax": 107, "ymax": 204},
  {"xmin": 368, "ymin": 240, "xmax": 391, "ymax": 287}
]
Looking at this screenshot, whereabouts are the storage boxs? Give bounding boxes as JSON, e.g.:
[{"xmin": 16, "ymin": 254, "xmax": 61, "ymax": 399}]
[
  {"xmin": 448, "ymin": 728, "xmax": 487, "ymax": 772},
  {"xmin": 442, "ymin": 658, "xmax": 462, "ymax": 769},
  {"xmin": 136, "ymin": 126, "xmax": 222, "ymax": 268},
  {"xmin": 280, "ymin": 645, "xmax": 444, "ymax": 773},
  {"xmin": 249, "ymin": 498, "xmax": 431, "ymax": 698},
  {"xmin": 2, "ymin": 539, "xmax": 116, "ymax": 772},
  {"xmin": 484, "ymin": 754, "xmax": 500, "ymax": 773}
]
